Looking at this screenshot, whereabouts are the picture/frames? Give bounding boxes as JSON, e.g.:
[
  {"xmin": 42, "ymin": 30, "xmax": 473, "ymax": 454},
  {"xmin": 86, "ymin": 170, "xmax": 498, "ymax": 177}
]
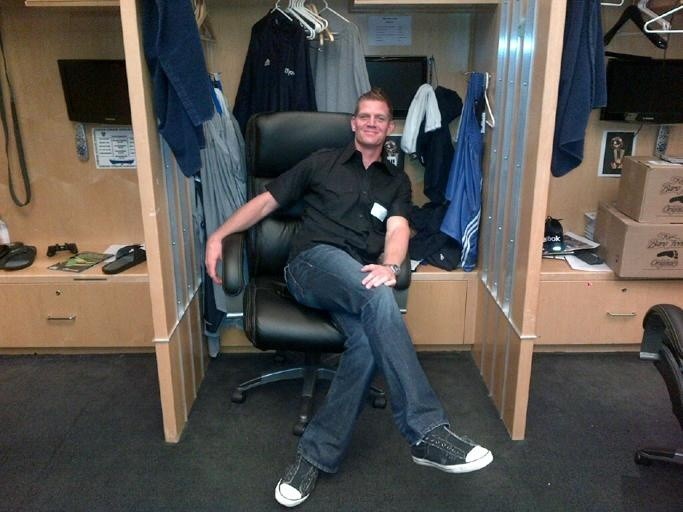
[{"xmin": 598, "ymin": 130, "xmax": 638, "ymax": 177}]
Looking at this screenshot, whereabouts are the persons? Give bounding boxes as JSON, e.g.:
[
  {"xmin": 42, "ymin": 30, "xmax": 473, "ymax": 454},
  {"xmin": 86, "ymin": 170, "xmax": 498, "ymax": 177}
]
[
  {"xmin": 606, "ymin": 136, "xmax": 626, "ymax": 171},
  {"xmin": 205, "ymin": 87, "xmax": 494, "ymax": 508}
]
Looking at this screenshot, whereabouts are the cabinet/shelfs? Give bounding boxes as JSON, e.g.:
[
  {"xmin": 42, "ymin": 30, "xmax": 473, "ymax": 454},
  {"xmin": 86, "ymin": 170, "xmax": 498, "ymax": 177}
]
[
  {"xmin": 534, "ymin": 250, "xmax": 681, "ymax": 355},
  {"xmin": 0, "ymin": 252, "xmax": 158, "ymax": 356},
  {"xmin": 208, "ymin": 261, "xmax": 477, "ymax": 355}
]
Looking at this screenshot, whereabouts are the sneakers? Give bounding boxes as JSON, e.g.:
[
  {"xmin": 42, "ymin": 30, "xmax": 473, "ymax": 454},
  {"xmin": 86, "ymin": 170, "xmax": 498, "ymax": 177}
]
[
  {"xmin": 274, "ymin": 455, "xmax": 319, "ymax": 507},
  {"xmin": 412, "ymin": 427, "xmax": 494, "ymax": 474}
]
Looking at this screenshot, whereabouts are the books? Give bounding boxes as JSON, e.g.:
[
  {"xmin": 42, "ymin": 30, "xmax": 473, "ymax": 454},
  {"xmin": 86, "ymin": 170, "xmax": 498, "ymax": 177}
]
[{"xmin": 47, "ymin": 251, "xmax": 114, "ymax": 274}]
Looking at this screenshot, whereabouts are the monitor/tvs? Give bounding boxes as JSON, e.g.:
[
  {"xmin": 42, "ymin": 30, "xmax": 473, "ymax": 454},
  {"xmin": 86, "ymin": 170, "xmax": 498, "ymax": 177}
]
[
  {"xmin": 601, "ymin": 56, "xmax": 683, "ymax": 126},
  {"xmin": 57, "ymin": 58, "xmax": 132, "ymax": 126},
  {"xmin": 363, "ymin": 55, "xmax": 429, "ymax": 120}
]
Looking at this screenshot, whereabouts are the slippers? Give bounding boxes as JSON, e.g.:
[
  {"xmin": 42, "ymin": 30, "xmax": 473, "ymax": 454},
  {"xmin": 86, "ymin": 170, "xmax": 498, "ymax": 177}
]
[
  {"xmin": 2, "ymin": 246, "xmax": 36, "ymax": 269},
  {"xmin": 102, "ymin": 245, "xmax": 147, "ymax": 274},
  {"xmin": 0, "ymin": 241, "xmax": 24, "ymax": 258}
]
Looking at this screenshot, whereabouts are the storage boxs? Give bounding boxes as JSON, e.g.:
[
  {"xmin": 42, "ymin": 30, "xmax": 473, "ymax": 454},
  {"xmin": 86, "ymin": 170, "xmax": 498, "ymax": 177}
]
[
  {"xmin": 592, "ymin": 199, "xmax": 681, "ymax": 279},
  {"xmin": 615, "ymin": 154, "xmax": 683, "ymax": 224}
]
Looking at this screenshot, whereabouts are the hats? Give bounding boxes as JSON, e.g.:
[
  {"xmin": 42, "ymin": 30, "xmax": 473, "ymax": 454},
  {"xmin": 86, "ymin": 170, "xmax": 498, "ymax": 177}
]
[{"xmin": 544, "ymin": 218, "xmax": 565, "ymax": 252}]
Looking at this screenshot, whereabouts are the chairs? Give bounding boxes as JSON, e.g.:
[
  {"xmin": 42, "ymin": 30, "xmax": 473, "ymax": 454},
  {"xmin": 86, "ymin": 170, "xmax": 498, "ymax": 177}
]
[
  {"xmin": 634, "ymin": 303, "xmax": 683, "ymax": 465},
  {"xmin": 230, "ymin": 111, "xmax": 389, "ymax": 435}
]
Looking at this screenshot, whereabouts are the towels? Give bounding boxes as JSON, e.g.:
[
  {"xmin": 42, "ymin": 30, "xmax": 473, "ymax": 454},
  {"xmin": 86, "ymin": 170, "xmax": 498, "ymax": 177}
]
[
  {"xmin": 400, "ymin": 85, "xmax": 442, "ymax": 154},
  {"xmin": 550, "ymin": 0, "xmax": 608, "ymax": 178}
]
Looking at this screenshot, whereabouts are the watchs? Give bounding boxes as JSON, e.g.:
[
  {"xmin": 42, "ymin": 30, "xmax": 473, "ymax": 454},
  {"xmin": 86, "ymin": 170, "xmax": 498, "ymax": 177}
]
[{"xmin": 382, "ymin": 261, "xmax": 400, "ymax": 278}]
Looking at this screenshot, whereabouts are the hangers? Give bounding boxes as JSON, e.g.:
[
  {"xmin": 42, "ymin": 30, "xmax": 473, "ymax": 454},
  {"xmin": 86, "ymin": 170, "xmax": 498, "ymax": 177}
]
[
  {"xmin": 271, "ymin": 0, "xmax": 350, "ymax": 54},
  {"xmin": 605, "ymin": 0, "xmax": 668, "ymax": 51},
  {"xmin": 643, "ymin": 5, "xmax": 683, "ymax": 34},
  {"xmin": 479, "ymin": 70, "xmax": 495, "ymax": 129},
  {"xmin": 615, "ymin": 0, "xmax": 669, "ymax": 38},
  {"xmin": 600, "ymin": 0, "xmax": 624, "ymax": 8},
  {"xmin": 635, "ymin": 0, "xmax": 673, "ymax": 36},
  {"xmin": 190, "ymin": 0, "xmax": 217, "ymax": 44}
]
